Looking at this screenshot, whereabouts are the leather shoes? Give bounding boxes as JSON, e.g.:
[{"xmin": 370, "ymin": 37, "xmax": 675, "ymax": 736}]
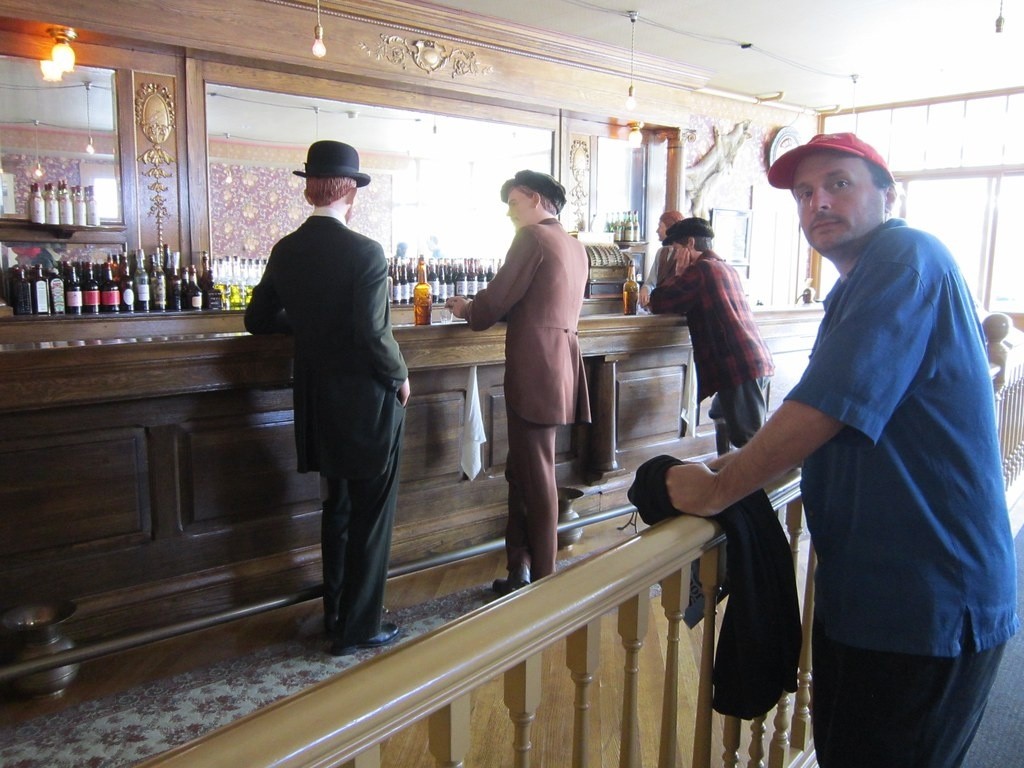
[
  {"xmin": 330, "ymin": 623, "xmax": 399, "ymax": 656},
  {"xmin": 324, "ymin": 612, "xmax": 339, "ymax": 639}
]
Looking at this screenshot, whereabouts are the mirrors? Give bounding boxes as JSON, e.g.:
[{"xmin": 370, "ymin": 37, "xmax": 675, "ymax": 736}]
[
  {"xmin": 199, "ymin": 81, "xmax": 553, "ymax": 301},
  {"xmin": 0, "ymin": 54, "xmax": 124, "ymax": 230},
  {"xmin": 596, "ymin": 134, "xmax": 647, "ymax": 242}
]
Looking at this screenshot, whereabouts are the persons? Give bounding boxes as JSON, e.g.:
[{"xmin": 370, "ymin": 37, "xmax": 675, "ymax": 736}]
[
  {"xmin": 445, "ymin": 169, "xmax": 592, "ymax": 594},
  {"xmin": 648, "ymin": 218, "xmax": 775, "ymax": 448},
  {"xmin": 638, "ymin": 210, "xmax": 684, "ymax": 310},
  {"xmin": 245, "ymin": 140, "xmax": 409, "ymax": 656},
  {"xmin": 664, "ymin": 131, "xmax": 1019, "ymax": 768}
]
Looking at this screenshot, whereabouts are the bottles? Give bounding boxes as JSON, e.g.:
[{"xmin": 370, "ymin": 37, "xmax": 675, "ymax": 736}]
[
  {"xmin": 163, "ymin": 244, "xmax": 171, "ymax": 273},
  {"xmin": 49, "ymin": 268, "xmax": 65, "ymax": 314},
  {"xmin": 120, "ymin": 252, "xmax": 134, "ymax": 312},
  {"xmin": 28, "ymin": 180, "xmax": 100, "ymax": 227},
  {"xmin": 202, "ymin": 251, "xmax": 213, "ymax": 310},
  {"xmin": 33, "ymin": 264, "xmax": 50, "ymax": 315},
  {"xmin": 187, "ymin": 265, "xmax": 202, "ymax": 310},
  {"xmin": 100, "ymin": 262, "xmax": 120, "ymax": 312},
  {"xmin": 623, "ymin": 260, "xmax": 638, "ymax": 315},
  {"xmin": 211, "ymin": 255, "xmax": 266, "ymax": 307},
  {"xmin": 182, "ymin": 267, "xmax": 189, "ymax": 309},
  {"xmin": 147, "ymin": 254, "xmax": 166, "ymax": 311},
  {"xmin": 605, "ymin": 210, "xmax": 640, "ymax": 242},
  {"xmin": 387, "ymin": 257, "xmax": 502, "ymax": 303},
  {"xmin": 65, "ymin": 267, "xmax": 83, "ymax": 314},
  {"xmin": 134, "ymin": 249, "xmax": 149, "ymax": 311},
  {"xmin": 166, "ymin": 252, "xmax": 181, "ymax": 311},
  {"xmin": 15, "ymin": 268, "xmax": 33, "ymax": 314},
  {"xmin": 83, "ymin": 262, "xmax": 100, "ymax": 313},
  {"xmin": 414, "ymin": 259, "xmax": 432, "ymax": 325}
]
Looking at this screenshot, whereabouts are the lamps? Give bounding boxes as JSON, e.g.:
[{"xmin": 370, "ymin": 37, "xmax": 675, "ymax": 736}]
[
  {"xmin": 40, "ymin": 60, "xmax": 63, "ymax": 82},
  {"xmin": 627, "ymin": 121, "xmax": 644, "ymax": 149},
  {"xmin": 46, "ymin": 26, "xmax": 79, "ymax": 73}
]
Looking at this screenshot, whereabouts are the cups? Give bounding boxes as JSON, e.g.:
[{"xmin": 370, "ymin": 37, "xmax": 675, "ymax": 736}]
[{"xmin": 440, "ymin": 309, "xmax": 453, "ymax": 323}]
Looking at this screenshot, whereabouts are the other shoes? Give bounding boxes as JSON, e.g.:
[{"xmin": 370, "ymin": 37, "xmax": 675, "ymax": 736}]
[{"xmin": 492, "ymin": 578, "xmax": 525, "ymax": 595}]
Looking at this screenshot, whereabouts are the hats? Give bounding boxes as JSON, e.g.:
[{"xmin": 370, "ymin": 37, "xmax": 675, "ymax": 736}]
[
  {"xmin": 662, "ymin": 217, "xmax": 714, "ymax": 245},
  {"xmin": 660, "ymin": 211, "xmax": 683, "ymax": 228},
  {"xmin": 292, "ymin": 141, "xmax": 371, "ymax": 188},
  {"xmin": 769, "ymin": 133, "xmax": 895, "ymax": 190},
  {"xmin": 515, "ymin": 169, "xmax": 566, "ymax": 215}
]
